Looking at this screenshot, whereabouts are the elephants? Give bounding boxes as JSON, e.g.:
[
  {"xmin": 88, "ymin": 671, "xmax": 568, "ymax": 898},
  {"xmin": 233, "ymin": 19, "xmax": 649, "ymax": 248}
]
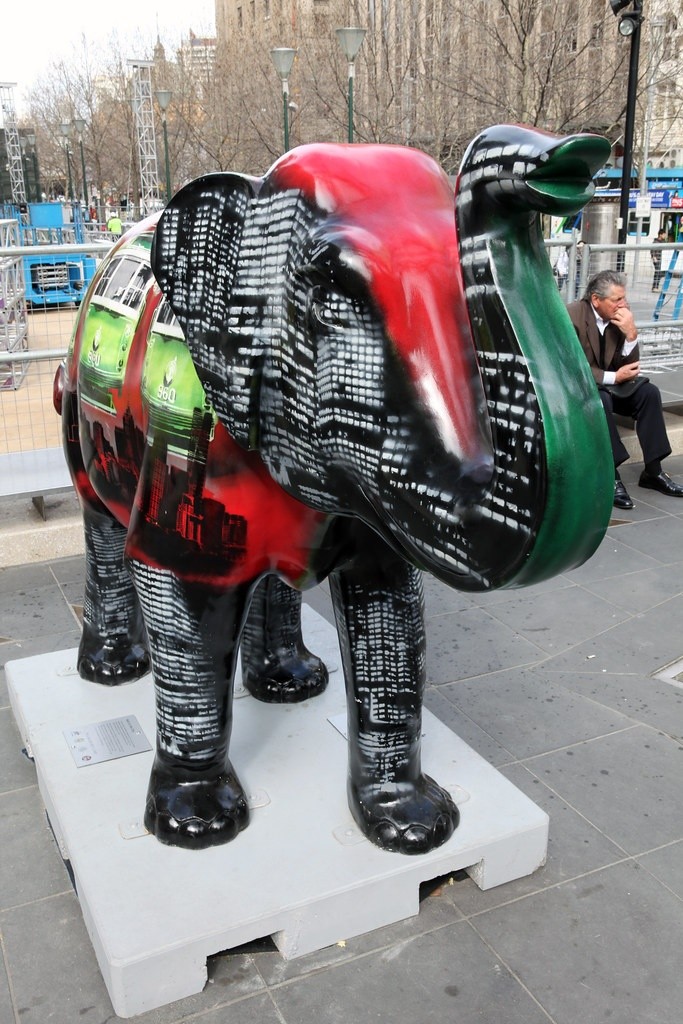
[{"xmin": 52, "ymin": 125, "xmax": 612, "ymax": 858}]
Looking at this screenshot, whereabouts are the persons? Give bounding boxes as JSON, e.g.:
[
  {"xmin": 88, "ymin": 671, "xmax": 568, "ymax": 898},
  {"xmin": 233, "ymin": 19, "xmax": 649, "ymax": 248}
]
[
  {"xmin": 565, "ymin": 271, "xmax": 683, "ymax": 508},
  {"xmin": 108, "ymin": 213, "xmax": 122, "ymax": 241},
  {"xmin": 650, "ymin": 229, "xmax": 666, "ymax": 292},
  {"xmin": 557, "ymin": 245, "xmax": 580, "ymax": 290}
]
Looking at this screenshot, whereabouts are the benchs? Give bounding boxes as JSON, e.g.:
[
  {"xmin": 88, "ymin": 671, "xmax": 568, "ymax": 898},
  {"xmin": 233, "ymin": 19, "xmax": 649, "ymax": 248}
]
[
  {"xmin": 613, "ymin": 372, "xmax": 683, "ymax": 431},
  {"xmin": 0, "ymin": 446, "xmax": 76, "ymax": 522}
]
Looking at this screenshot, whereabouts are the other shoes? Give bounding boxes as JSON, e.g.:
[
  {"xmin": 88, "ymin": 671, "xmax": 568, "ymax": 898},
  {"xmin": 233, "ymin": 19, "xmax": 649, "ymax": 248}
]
[{"xmin": 652, "ymin": 288, "xmax": 660, "ymax": 293}]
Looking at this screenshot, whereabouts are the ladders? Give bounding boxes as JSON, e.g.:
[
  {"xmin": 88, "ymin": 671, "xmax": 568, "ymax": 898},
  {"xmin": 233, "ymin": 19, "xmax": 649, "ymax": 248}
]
[{"xmin": 652, "ymin": 233, "xmax": 683, "ymax": 322}]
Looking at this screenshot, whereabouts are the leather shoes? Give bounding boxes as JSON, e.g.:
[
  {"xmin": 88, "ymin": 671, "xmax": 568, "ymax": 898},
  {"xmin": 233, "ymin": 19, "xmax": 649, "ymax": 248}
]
[
  {"xmin": 638, "ymin": 470, "xmax": 683, "ymax": 497},
  {"xmin": 611, "ymin": 479, "xmax": 633, "ymax": 508}
]
